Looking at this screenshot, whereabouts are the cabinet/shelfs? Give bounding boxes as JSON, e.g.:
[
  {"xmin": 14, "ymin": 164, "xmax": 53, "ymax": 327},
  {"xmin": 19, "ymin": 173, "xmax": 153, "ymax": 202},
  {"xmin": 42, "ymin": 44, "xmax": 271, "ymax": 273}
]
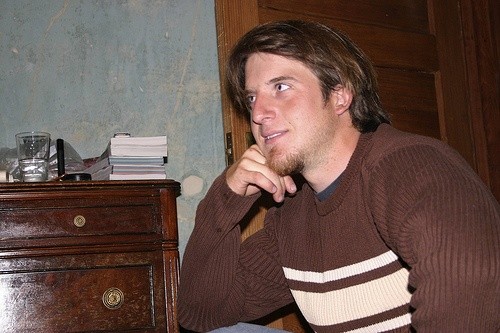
[{"xmin": 0, "ymin": 179, "xmax": 181, "ymax": 333}]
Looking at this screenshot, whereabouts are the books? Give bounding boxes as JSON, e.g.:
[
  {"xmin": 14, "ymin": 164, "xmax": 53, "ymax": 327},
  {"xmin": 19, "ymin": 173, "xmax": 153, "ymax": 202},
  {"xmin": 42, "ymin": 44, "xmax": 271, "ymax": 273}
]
[{"xmin": 83, "ymin": 135, "xmax": 167, "ymax": 180}]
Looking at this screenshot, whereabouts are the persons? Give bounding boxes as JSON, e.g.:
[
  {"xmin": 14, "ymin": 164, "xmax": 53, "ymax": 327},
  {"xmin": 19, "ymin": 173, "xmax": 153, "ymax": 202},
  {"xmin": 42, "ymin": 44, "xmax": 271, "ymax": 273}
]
[{"xmin": 175, "ymin": 18, "xmax": 499, "ymax": 333}]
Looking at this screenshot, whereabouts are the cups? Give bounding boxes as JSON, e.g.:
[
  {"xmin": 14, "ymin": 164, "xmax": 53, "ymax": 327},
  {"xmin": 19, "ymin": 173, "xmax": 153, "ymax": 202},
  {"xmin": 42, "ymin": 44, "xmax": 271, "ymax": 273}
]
[{"xmin": 15, "ymin": 132, "xmax": 51, "ymax": 182}]
